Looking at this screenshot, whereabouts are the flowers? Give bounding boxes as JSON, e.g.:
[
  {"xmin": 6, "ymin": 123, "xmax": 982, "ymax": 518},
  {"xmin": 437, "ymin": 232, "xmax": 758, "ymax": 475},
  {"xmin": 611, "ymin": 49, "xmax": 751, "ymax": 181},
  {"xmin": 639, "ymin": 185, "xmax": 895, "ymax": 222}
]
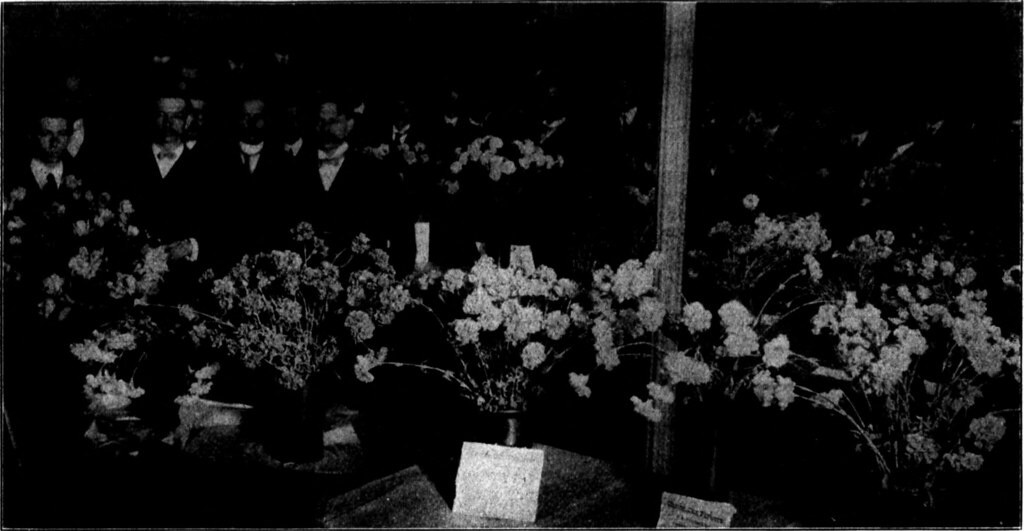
[{"xmin": 3, "ymin": 173, "xmax": 1021, "ymax": 530}]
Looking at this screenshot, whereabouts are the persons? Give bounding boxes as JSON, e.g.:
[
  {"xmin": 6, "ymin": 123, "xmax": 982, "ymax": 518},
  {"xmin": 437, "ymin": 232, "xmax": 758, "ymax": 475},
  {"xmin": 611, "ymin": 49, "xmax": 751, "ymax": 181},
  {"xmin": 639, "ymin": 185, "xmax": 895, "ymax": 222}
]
[{"xmin": 2, "ymin": 82, "xmax": 412, "ymax": 267}]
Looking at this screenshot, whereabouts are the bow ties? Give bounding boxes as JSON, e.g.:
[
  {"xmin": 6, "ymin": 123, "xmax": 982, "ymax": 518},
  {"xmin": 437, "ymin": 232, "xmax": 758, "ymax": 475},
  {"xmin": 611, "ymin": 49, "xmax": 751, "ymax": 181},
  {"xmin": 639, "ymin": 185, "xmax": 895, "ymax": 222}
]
[
  {"xmin": 316, "ymin": 158, "xmax": 340, "ymax": 168},
  {"xmin": 158, "ymin": 152, "xmax": 174, "ymax": 160}
]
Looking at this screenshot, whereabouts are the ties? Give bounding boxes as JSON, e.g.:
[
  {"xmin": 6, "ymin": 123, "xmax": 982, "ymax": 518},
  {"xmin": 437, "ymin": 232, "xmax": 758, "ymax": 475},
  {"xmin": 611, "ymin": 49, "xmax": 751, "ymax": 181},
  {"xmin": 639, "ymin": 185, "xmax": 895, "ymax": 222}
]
[
  {"xmin": 43, "ymin": 173, "xmax": 58, "ymax": 193},
  {"xmin": 241, "ymin": 149, "xmax": 261, "ymax": 176}
]
